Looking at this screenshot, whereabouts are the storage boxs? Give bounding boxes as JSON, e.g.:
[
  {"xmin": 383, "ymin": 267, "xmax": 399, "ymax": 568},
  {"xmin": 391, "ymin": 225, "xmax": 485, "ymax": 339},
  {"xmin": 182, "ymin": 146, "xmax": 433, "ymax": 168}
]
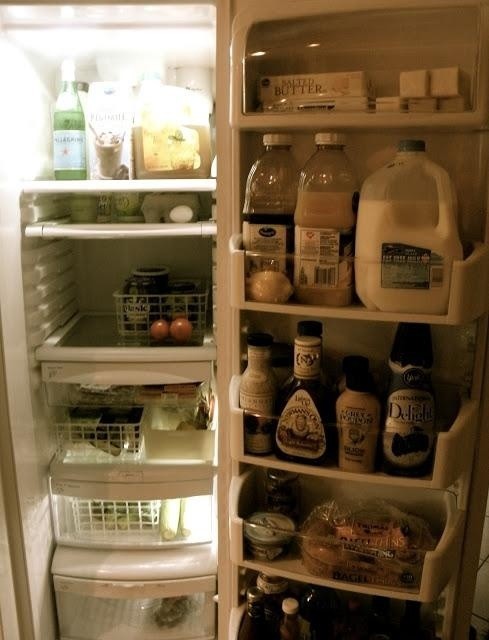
[{"xmin": 52, "ymin": 575, "xmax": 217, "ymax": 640}]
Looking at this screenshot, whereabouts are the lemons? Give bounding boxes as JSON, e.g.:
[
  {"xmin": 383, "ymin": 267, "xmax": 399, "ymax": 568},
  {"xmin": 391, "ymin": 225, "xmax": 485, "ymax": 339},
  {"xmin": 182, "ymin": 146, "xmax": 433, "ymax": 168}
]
[{"xmin": 244, "ymin": 270, "xmax": 294, "ymax": 304}]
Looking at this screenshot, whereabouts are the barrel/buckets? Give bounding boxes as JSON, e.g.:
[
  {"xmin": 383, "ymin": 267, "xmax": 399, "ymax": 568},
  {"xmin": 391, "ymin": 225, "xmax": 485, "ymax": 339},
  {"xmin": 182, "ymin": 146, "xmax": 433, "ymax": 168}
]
[{"xmin": 353, "ymin": 140, "xmax": 464, "ymax": 316}]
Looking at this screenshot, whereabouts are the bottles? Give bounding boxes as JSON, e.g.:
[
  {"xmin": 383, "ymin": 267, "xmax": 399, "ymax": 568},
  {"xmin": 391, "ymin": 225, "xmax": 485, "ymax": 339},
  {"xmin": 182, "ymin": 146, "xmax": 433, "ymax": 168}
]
[
  {"xmin": 51, "ymin": 82, "xmax": 86, "ymax": 179},
  {"xmin": 238, "ymin": 460, "xmax": 299, "ymax": 568},
  {"xmin": 239, "ymin": 131, "xmax": 296, "ymax": 301},
  {"xmin": 239, "ymin": 321, "xmax": 437, "ymax": 485},
  {"xmin": 296, "ymin": 130, "xmax": 360, "ymax": 304},
  {"xmin": 239, "ymin": 572, "xmax": 436, "ymax": 639}
]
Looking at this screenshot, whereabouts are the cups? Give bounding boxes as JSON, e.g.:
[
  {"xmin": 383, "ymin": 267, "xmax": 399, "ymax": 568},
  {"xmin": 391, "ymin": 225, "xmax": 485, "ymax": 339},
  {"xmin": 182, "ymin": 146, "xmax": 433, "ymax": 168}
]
[{"xmin": 94, "ymin": 131, "xmax": 124, "ymax": 179}]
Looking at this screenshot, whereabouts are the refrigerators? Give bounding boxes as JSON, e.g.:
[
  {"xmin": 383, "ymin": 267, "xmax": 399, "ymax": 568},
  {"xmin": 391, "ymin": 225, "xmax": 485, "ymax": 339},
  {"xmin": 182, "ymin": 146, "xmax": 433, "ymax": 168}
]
[{"xmin": 1, "ymin": 0, "xmax": 489, "ymax": 640}]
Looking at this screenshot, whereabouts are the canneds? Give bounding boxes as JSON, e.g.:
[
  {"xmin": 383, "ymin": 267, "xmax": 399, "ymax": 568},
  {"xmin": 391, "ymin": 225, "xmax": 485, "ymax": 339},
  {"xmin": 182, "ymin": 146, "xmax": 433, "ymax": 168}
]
[
  {"xmin": 244, "ymin": 513, "xmax": 294, "ymax": 560},
  {"xmin": 123, "ymin": 275, "xmax": 152, "ymax": 331}
]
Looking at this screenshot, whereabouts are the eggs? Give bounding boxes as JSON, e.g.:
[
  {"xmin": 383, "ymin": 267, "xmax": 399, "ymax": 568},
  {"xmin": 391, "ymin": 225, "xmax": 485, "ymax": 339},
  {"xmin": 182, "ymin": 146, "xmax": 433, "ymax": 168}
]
[
  {"xmin": 168, "ymin": 204, "xmax": 194, "ymax": 223},
  {"xmin": 169, "ymin": 316, "xmax": 194, "ymax": 343},
  {"xmin": 149, "ymin": 319, "xmax": 169, "ymax": 341}
]
